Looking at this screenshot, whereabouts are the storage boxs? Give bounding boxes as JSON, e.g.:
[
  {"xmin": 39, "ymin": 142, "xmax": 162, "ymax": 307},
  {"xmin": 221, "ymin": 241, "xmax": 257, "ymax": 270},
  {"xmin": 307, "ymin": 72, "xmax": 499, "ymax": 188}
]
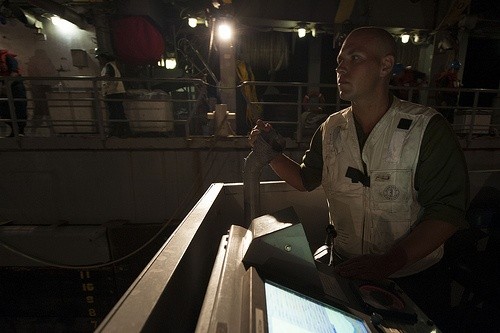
[
  {"xmin": 207, "ymin": 113, "xmax": 236, "ymax": 135},
  {"xmin": 123, "ymin": 91, "xmax": 175, "ymax": 134},
  {"xmin": 452, "ymin": 110, "xmax": 492, "ymax": 135}
]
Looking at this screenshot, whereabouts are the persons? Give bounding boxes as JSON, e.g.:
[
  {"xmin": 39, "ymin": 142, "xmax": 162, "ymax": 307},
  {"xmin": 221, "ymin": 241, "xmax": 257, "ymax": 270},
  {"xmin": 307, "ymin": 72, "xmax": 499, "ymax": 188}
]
[
  {"xmin": 250, "ymin": 27, "xmax": 468, "ymax": 319},
  {"xmin": 95, "ymin": 54, "xmax": 129, "ymax": 137},
  {"xmin": 0, "ymin": 50, "xmax": 28, "ymax": 138}
]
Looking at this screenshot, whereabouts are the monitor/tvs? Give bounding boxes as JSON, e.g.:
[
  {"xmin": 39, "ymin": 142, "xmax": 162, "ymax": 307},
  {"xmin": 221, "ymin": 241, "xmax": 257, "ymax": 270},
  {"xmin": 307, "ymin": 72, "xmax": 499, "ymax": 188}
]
[{"xmin": 262, "ymin": 278, "xmax": 372, "ymax": 333}]
